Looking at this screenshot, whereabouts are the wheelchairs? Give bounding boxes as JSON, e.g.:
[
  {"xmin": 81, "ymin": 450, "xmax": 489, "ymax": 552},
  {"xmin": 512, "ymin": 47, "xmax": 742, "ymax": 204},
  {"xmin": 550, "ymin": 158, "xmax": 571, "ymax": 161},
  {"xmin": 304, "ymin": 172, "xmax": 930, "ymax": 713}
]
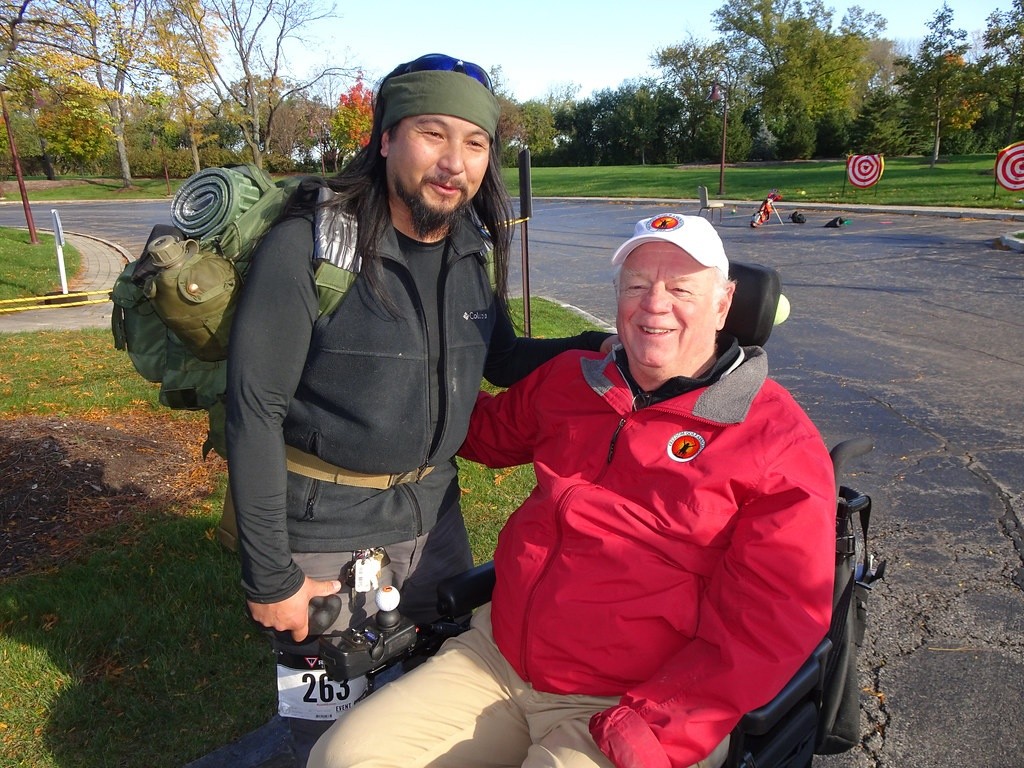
[{"xmin": 309, "ymin": 434, "xmax": 880, "ymax": 768}]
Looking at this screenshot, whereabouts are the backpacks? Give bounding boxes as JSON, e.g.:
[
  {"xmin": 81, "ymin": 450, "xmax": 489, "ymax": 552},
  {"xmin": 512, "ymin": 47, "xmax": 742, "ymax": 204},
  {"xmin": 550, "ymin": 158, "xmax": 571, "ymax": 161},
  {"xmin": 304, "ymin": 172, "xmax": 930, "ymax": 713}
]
[{"xmin": 108, "ymin": 161, "xmax": 497, "ymax": 488}]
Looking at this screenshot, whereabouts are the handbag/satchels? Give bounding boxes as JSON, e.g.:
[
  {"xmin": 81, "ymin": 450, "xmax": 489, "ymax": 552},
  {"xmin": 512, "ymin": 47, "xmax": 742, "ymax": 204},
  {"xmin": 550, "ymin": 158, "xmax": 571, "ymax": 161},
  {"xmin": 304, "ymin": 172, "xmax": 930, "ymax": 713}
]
[{"xmin": 815, "ymin": 495, "xmax": 886, "ymax": 755}]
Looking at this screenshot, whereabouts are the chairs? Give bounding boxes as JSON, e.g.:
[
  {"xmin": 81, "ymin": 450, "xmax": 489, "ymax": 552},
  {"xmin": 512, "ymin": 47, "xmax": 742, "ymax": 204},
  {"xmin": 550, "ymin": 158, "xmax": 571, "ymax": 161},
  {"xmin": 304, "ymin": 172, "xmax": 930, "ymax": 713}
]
[{"xmin": 698, "ymin": 185, "xmax": 725, "ymax": 225}]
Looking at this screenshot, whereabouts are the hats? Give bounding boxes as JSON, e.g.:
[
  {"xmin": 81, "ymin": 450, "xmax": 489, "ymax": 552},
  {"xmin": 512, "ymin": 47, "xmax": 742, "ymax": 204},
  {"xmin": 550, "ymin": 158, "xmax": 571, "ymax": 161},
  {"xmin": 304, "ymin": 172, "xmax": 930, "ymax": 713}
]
[{"xmin": 611, "ymin": 213, "xmax": 729, "ymax": 282}]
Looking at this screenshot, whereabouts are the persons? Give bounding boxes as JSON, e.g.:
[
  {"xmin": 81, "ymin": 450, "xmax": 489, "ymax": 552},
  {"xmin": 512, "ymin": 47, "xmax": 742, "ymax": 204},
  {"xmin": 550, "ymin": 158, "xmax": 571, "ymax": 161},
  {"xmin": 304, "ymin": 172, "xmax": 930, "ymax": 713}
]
[
  {"xmin": 306, "ymin": 213, "xmax": 837, "ymax": 768},
  {"xmin": 227, "ymin": 53, "xmax": 621, "ymax": 768}
]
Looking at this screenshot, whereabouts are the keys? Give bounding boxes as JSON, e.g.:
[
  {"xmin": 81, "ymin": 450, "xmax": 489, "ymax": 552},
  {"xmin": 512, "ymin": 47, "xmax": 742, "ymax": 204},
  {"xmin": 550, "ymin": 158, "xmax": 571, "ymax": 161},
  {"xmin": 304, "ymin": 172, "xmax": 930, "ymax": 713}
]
[{"xmin": 346, "ymin": 546, "xmax": 390, "ymax": 612}]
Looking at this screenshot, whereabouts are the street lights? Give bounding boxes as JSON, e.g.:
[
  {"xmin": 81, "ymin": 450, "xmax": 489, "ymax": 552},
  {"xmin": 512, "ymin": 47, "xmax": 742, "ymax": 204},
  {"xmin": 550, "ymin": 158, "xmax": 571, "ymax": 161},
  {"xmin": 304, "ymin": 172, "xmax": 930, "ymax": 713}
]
[{"xmin": 708, "ymin": 67, "xmax": 731, "ymax": 196}]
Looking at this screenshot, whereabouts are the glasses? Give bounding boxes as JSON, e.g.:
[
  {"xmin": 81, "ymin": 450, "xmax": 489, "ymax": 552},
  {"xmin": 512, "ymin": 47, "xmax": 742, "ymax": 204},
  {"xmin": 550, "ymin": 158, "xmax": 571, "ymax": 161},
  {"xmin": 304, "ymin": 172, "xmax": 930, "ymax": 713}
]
[{"xmin": 402, "ymin": 53, "xmax": 494, "ymax": 95}]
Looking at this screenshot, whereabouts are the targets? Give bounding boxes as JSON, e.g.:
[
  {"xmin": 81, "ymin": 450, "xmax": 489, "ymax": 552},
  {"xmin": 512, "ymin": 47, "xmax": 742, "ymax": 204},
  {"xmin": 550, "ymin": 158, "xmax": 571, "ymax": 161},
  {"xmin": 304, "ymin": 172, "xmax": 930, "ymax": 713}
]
[
  {"xmin": 842, "ymin": 152, "xmax": 885, "ymax": 189},
  {"xmin": 993, "ymin": 141, "xmax": 1024, "ymax": 191}
]
